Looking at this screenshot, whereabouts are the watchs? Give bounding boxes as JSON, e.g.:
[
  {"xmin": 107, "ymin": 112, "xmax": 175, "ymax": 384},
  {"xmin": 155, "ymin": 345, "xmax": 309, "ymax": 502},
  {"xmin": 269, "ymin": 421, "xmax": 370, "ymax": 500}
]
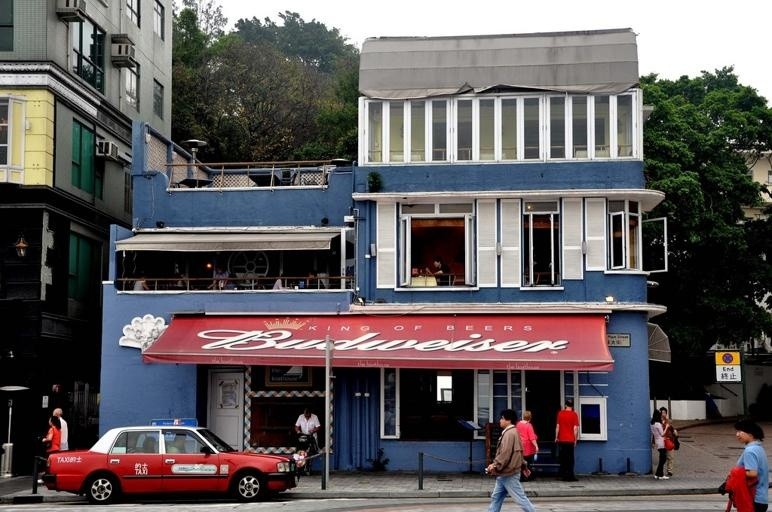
[{"xmin": 493, "ymin": 463, "xmax": 497, "ymax": 471}]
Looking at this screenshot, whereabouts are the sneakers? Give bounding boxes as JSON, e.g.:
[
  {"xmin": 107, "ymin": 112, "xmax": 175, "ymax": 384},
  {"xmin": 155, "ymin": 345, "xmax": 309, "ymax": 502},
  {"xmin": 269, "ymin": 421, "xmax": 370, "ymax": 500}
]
[{"xmin": 653, "ymin": 475, "xmax": 670, "ymax": 480}]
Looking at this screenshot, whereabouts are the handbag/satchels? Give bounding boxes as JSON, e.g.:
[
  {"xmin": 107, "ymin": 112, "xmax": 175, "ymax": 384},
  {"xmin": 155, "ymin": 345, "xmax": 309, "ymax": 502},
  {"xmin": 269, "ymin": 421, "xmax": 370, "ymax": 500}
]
[{"xmin": 663, "ymin": 436, "xmax": 679, "ymax": 450}]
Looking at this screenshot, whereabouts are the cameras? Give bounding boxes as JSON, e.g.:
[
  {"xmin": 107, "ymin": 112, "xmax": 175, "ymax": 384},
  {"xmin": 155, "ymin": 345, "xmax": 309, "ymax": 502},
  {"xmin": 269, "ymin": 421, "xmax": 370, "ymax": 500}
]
[{"xmin": 485, "ymin": 465, "xmax": 497, "ymax": 475}]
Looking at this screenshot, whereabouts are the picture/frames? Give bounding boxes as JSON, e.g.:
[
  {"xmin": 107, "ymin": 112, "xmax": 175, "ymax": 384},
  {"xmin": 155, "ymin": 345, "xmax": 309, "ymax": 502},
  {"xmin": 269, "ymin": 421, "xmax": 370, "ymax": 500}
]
[
  {"xmin": 606, "ymin": 333, "xmax": 631, "ymax": 348},
  {"xmin": 265, "ymin": 366, "xmax": 313, "ymax": 388},
  {"xmin": 217, "ymin": 379, "xmax": 239, "ymax": 409}
]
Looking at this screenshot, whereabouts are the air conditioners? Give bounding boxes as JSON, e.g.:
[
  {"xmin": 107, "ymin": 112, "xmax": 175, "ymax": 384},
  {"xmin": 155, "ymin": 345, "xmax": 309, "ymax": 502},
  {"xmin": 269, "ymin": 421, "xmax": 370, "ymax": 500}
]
[
  {"xmin": 111, "ymin": 43, "xmax": 135, "ymax": 57},
  {"xmin": 56, "ymin": 0, "xmax": 87, "ymax": 12},
  {"xmin": 96, "ymin": 141, "xmax": 119, "ymax": 162}
]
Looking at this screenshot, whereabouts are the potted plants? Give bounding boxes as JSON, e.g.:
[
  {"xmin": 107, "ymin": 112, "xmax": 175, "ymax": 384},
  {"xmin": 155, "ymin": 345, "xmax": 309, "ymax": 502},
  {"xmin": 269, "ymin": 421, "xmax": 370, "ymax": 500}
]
[{"xmin": 368, "ymin": 445, "xmax": 389, "ymax": 473}]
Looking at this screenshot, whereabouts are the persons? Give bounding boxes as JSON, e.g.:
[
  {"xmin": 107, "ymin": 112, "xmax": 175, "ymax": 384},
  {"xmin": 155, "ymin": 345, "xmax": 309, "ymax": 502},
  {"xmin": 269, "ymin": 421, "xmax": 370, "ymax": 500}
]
[
  {"xmin": 486, "ymin": 409, "xmax": 537, "ymax": 512},
  {"xmin": 272, "ymin": 272, "xmax": 290, "ymax": 290},
  {"xmin": 515, "ymin": 410, "xmax": 539, "ymax": 482},
  {"xmin": 650, "ymin": 409, "xmax": 671, "ymax": 480},
  {"xmin": 52, "ymin": 408, "xmax": 69, "ymax": 452},
  {"xmin": 42, "ymin": 415, "xmax": 61, "ymax": 459},
  {"xmin": 305, "ymin": 270, "xmax": 325, "ymax": 289},
  {"xmin": 166, "ymin": 433, "xmax": 186, "ymax": 454},
  {"xmin": 133, "ymin": 273, "xmax": 149, "ymax": 290},
  {"xmin": 659, "ymin": 407, "xmax": 678, "ymax": 476},
  {"xmin": 426, "ymin": 256, "xmax": 452, "ymax": 286},
  {"xmin": 140, "ymin": 437, "xmax": 157, "ymax": 453},
  {"xmin": 207, "ymin": 266, "xmax": 230, "ymax": 289},
  {"xmin": 295, "ymin": 450, "xmax": 305, "ymax": 468},
  {"xmin": 294, "ymin": 407, "xmax": 321, "ymax": 453},
  {"xmin": 721, "ymin": 420, "xmax": 769, "ymax": 512},
  {"xmin": 554, "ymin": 398, "xmax": 580, "ymax": 481}
]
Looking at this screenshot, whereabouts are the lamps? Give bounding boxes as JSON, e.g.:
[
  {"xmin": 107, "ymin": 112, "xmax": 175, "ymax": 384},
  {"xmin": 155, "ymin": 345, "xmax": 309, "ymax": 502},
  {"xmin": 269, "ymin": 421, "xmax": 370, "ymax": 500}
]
[{"xmin": 14, "ymin": 231, "xmax": 28, "ymax": 258}]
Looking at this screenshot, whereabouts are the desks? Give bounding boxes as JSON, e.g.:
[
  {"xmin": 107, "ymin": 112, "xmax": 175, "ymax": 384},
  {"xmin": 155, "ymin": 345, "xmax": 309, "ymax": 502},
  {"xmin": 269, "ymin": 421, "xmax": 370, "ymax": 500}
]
[
  {"xmin": 525, "ymin": 271, "xmax": 558, "ymax": 285},
  {"xmin": 420, "ymin": 273, "xmax": 455, "ymax": 286}
]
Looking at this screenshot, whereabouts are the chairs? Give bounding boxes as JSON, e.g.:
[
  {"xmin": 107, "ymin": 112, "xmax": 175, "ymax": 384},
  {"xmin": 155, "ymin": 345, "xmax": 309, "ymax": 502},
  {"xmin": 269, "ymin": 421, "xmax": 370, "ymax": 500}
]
[
  {"xmin": 452, "ymin": 275, "xmax": 465, "ymax": 285},
  {"xmin": 175, "ymin": 178, "xmax": 212, "ymax": 190},
  {"xmin": 247, "ymin": 173, "xmax": 292, "ymax": 186},
  {"xmin": 411, "ymin": 277, "xmax": 438, "ymax": 286}
]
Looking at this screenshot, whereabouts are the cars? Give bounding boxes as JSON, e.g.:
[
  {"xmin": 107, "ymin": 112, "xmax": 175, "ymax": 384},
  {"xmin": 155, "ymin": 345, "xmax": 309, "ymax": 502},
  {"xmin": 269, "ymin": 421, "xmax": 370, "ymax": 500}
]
[{"xmin": 41, "ymin": 426, "xmax": 299, "ymax": 503}]
